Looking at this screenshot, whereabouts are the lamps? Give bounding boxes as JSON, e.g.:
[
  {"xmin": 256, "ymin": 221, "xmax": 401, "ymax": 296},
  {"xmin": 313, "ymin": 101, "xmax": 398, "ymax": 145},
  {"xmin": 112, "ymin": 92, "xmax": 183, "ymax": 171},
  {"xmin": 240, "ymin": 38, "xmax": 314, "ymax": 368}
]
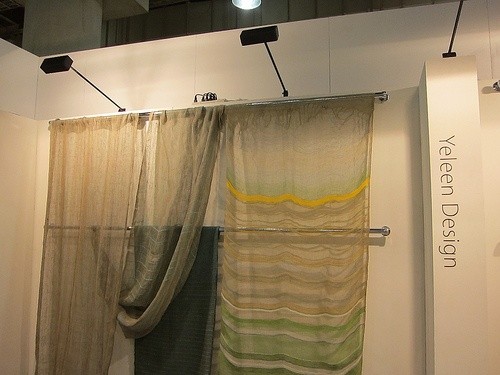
[
  {"xmin": 239, "ymin": 25, "xmax": 288, "ymax": 97},
  {"xmin": 38, "ymin": 55, "xmax": 127, "ymax": 112}
]
